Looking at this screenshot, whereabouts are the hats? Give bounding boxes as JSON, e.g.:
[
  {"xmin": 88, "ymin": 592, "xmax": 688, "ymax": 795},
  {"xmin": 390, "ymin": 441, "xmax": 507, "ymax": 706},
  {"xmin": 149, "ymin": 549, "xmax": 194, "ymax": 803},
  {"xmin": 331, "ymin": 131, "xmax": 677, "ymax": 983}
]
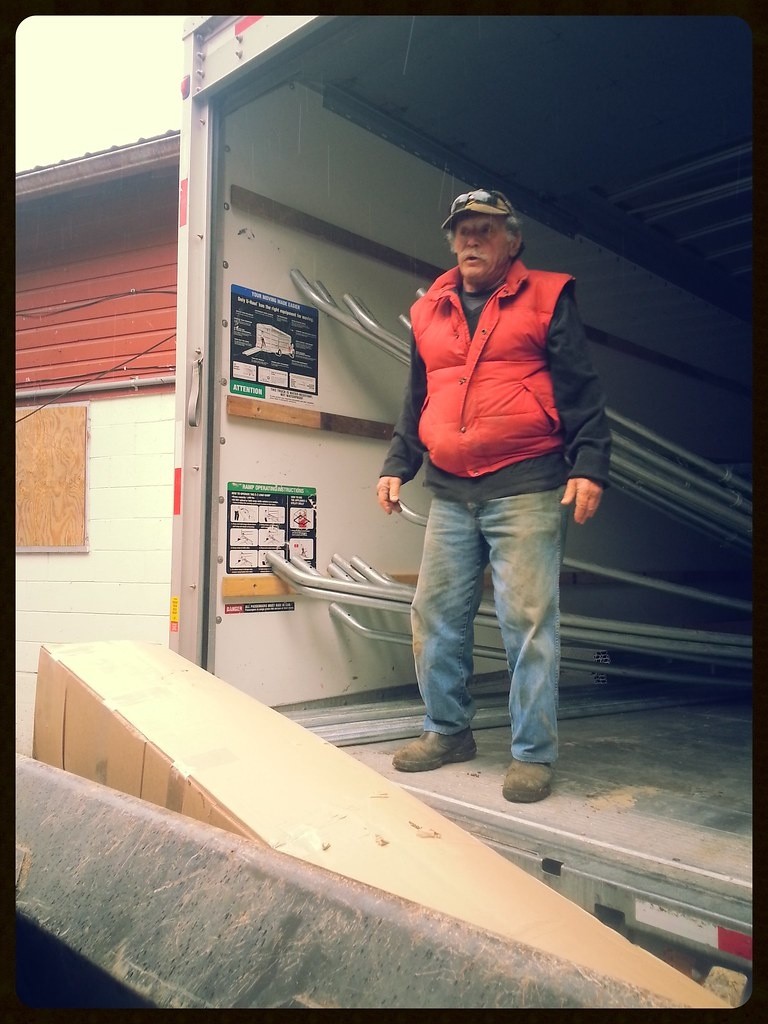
[{"xmin": 441, "ymin": 189, "xmax": 517, "ymax": 229}]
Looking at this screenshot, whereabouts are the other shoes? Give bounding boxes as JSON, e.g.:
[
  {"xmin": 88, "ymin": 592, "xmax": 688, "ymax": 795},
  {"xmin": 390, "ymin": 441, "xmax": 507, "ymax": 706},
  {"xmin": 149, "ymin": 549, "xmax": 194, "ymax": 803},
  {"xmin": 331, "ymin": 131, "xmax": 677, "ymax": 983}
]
[
  {"xmin": 392, "ymin": 725, "xmax": 475, "ymax": 771},
  {"xmin": 502, "ymin": 758, "xmax": 552, "ymax": 803}
]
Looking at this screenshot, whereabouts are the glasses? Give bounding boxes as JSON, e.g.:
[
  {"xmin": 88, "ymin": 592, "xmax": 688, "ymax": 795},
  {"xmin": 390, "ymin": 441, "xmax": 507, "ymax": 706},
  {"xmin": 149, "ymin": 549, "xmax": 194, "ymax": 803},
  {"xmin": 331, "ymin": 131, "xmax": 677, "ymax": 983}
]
[{"xmin": 450, "ymin": 191, "xmax": 501, "ymax": 210}]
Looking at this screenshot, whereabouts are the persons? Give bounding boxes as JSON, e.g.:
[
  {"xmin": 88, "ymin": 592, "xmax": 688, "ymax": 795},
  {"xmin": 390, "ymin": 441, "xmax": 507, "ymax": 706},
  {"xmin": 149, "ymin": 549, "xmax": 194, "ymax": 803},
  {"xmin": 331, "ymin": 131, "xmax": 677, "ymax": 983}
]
[{"xmin": 376, "ymin": 189, "xmax": 611, "ymax": 803}]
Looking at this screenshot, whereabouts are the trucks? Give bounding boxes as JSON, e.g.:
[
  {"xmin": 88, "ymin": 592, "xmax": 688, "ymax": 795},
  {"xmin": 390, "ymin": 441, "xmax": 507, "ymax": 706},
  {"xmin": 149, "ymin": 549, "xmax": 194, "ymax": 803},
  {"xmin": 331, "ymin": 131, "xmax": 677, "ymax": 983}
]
[{"xmin": 150, "ymin": 15, "xmax": 757, "ymax": 977}]
